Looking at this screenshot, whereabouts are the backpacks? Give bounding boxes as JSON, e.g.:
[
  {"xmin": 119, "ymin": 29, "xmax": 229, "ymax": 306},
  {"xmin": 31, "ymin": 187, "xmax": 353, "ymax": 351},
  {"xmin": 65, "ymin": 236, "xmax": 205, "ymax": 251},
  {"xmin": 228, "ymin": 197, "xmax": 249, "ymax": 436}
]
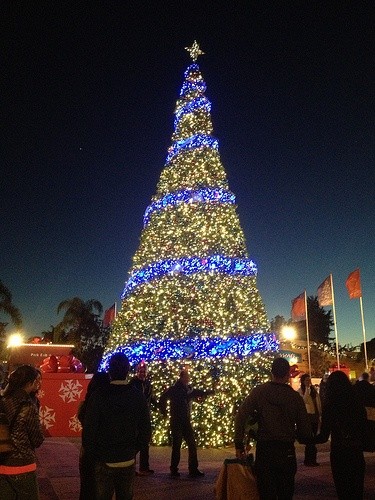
[{"xmin": 1, "ymin": 397, "xmax": 27, "ymax": 463}]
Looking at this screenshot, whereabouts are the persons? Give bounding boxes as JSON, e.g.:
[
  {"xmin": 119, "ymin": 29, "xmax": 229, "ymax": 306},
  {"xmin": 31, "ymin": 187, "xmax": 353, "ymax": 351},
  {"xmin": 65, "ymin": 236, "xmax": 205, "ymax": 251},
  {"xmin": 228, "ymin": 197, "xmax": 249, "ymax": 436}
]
[
  {"xmin": 320, "ymin": 366, "xmax": 375, "ymax": 452},
  {"xmin": 83, "ymin": 352, "xmax": 151, "ymax": 500},
  {"xmin": 294, "ymin": 373, "xmax": 321, "ymax": 466},
  {"xmin": 159, "ymin": 368, "xmax": 230, "ymax": 477},
  {"xmin": 0, "ymin": 365, "xmax": 44, "ymax": 500},
  {"xmin": 234, "ymin": 357, "xmax": 317, "ymax": 500},
  {"xmin": 130, "ymin": 360, "xmax": 168, "ymax": 474},
  {"xmin": 76, "ymin": 372, "xmax": 114, "ymax": 500},
  {"xmin": 312, "ymin": 370, "xmax": 367, "ymax": 500}
]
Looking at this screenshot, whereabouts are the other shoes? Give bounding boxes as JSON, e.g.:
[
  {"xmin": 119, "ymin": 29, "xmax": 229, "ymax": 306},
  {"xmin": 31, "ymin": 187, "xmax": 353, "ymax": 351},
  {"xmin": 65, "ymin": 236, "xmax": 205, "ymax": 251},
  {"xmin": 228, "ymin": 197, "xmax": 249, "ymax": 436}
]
[
  {"xmin": 304, "ymin": 459, "xmax": 320, "ymax": 467},
  {"xmin": 170, "ymin": 467, "xmax": 179, "ymax": 478},
  {"xmin": 139, "ymin": 469, "xmax": 155, "ymax": 476},
  {"xmin": 189, "ymin": 468, "xmax": 206, "ymax": 477}
]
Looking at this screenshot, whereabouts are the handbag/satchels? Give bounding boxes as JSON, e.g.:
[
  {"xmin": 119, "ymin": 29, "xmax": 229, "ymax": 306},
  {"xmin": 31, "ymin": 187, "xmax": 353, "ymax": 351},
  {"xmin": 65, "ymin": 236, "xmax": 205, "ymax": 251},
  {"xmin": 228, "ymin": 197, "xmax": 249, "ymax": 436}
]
[{"xmin": 214, "ymin": 453, "xmax": 258, "ymax": 500}]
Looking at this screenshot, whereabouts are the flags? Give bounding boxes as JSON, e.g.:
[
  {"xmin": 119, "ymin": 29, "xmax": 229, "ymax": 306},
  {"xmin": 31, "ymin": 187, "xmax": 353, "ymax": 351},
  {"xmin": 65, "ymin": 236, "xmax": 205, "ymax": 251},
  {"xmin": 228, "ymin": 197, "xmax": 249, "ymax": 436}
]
[
  {"xmin": 291, "ymin": 291, "xmax": 306, "ymax": 323},
  {"xmin": 346, "ymin": 269, "xmax": 362, "ymax": 300},
  {"xmin": 317, "ymin": 275, "xmax": 333, "ymax": 307},
  {"xmin": 103, "ymin": 303, "xmax": 115, "ymax": 326}
]
